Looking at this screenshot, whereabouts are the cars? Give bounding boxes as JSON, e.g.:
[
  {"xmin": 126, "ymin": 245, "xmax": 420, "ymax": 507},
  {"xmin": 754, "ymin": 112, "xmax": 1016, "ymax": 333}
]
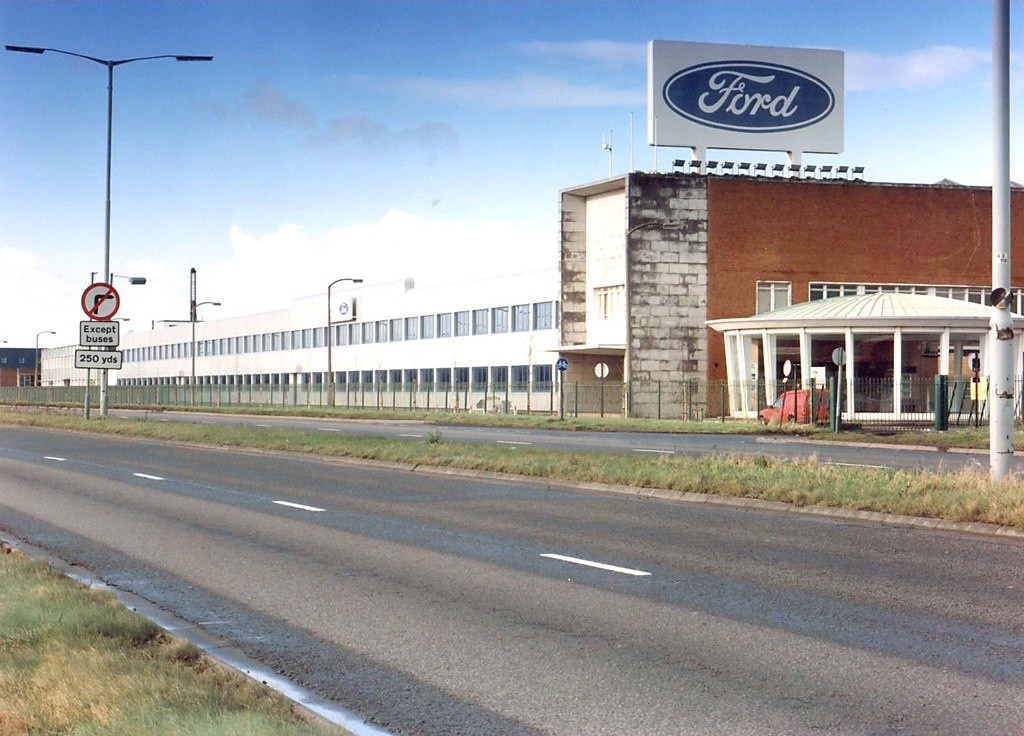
[{"xmin": 757, "ymin": 389, "xmax": 832, "ymax": 427}]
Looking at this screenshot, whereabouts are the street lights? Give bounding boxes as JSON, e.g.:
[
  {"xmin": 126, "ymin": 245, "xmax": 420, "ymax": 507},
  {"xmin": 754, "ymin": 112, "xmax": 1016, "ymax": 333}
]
[
  {"xmin": 327, "ymin": 277, "xmax": 364, "ymax": 409},
  {"xmin": 33, "ymin": 331, "xmax": 57, "ymax": 386},
  {"xmin": 190, "ymin": 301, "xmax": 221, "ymax": 406},
  {"xmin": 4, "ymin": 44, "xmax": 214, "ymax": 418}
]
